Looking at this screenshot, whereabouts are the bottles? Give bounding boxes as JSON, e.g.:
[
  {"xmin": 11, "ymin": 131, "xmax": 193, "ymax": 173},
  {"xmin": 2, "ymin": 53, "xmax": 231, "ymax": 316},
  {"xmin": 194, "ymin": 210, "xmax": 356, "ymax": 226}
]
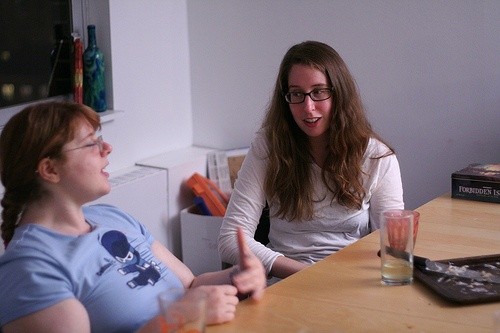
[{"xmin": 83, "ymin": 25, "xmax": 106, "ymax": 111}]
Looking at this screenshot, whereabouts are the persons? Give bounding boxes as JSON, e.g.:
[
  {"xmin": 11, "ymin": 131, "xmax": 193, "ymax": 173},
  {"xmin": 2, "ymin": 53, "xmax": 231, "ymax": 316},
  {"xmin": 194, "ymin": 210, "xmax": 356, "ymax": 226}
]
[
  {"xmin": 219, "ymin": 40, "xmax": 404, "ymax": 287},
  {"xmin": 0, "ymin": 101, "xmax": 266, "ymax": 333}
]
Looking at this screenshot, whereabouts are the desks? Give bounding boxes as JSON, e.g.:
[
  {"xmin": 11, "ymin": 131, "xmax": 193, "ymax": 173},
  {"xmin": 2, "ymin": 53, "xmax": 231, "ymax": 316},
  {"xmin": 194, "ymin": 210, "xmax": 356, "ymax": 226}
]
[{"xmin": 202, "ymin": 190, "xmax": 500, "ymax": 333}]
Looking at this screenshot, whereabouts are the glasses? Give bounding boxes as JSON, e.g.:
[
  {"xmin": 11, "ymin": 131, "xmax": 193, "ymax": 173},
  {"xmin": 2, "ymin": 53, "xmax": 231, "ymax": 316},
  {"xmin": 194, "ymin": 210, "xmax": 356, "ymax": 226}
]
[
  {"xmin": 47, "ymin": 135, "xmax": 106, "ymax": 156},
  {"xmin": 284, "ymin": 86, "xmax": 336, "ymax": 104}
]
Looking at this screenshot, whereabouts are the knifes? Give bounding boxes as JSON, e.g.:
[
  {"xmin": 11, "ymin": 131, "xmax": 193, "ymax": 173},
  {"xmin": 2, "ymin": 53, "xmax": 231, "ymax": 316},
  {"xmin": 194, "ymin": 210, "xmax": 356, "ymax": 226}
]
[{"xmin": 377, "ymin": 246, "xmax": 500, "ymax": 284}]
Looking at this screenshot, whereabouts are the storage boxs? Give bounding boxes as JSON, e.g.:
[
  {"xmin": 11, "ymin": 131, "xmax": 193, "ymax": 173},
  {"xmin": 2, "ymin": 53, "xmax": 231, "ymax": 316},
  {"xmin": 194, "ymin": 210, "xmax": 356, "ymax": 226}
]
[{"xmin": 451, "ymin": 163, "xmax": 500, "ymax": 203}]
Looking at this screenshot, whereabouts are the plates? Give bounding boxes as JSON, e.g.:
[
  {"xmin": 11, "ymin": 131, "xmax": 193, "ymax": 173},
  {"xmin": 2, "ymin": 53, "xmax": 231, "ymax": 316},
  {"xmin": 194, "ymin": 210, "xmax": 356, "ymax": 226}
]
[{"xmin": 414, "ymin": 254, "xmax": 500, "ymax": 302}]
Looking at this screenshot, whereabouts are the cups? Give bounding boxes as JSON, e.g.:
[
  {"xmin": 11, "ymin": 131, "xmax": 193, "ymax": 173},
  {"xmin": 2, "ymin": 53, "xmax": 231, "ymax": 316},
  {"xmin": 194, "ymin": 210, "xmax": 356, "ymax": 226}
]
[
  {"xmin": 383, "ymin": 211, "xmax": 420, "ymax": 251},
  {"xmin": 379, "ymin": 209, "xmax": 414, "ymax": 286}
]
